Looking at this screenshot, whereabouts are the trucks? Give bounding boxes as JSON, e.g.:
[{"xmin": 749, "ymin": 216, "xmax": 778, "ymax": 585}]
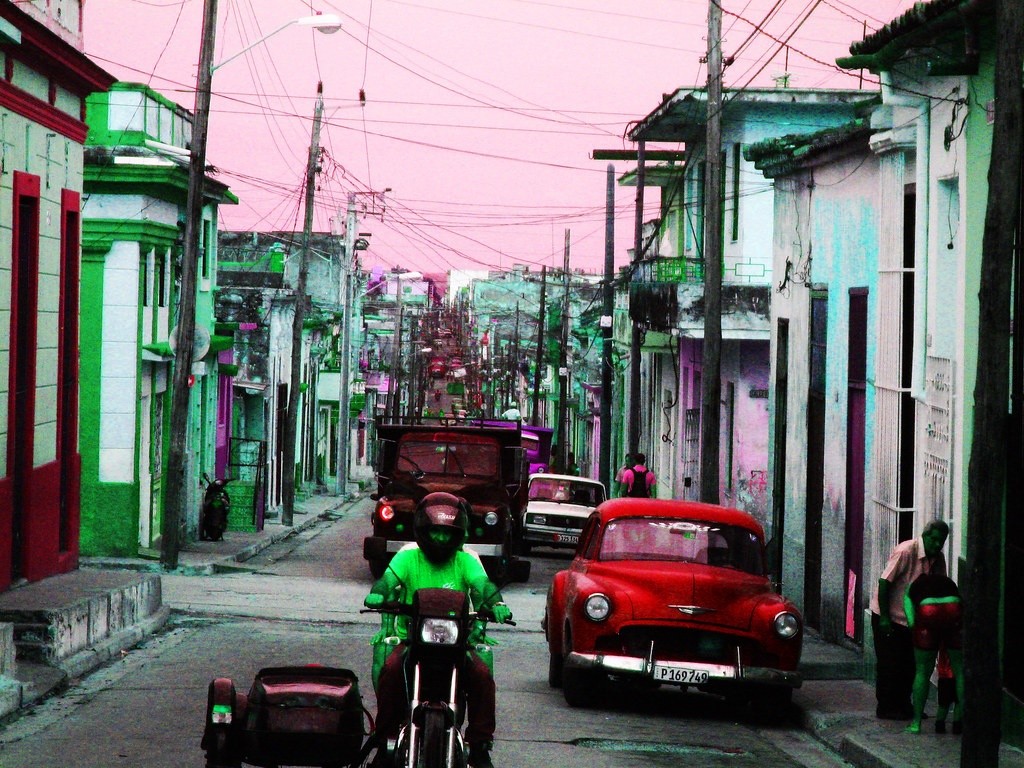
[
  {"xmin": 469, "ymin": 420, "xmax": 555, "ymax": 478},
  {"xmin": 361, "ymin": 413, "xmax": 531, "ymax": 581}
]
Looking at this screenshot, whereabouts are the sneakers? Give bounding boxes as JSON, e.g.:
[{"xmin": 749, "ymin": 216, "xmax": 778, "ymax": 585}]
[{"xmin": 465, "ymin": 738, "xmax": 493, "ymax": 768}]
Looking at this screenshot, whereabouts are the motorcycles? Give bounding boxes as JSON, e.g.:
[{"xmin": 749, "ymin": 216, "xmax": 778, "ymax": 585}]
[
  {"xmin": 202, "ymin": 535, "xmax": 534, "ymax": 768},
  {"xmin": 200, "ymin": 471, "xmax": 237, "ymax": 542}
]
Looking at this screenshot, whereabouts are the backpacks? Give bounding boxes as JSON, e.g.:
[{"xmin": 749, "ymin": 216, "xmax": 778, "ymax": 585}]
[{"xmin": 629, "ymin": 467, "xmax": 649, "ymax": 498}]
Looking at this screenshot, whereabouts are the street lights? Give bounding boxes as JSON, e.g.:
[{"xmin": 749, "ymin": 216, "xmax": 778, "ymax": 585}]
[{"xmin": 157, "ymin": 1, "xmax": 344, "ymax": 574}]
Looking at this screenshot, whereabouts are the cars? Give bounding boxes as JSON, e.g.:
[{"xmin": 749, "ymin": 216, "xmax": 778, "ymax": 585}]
[
  {"xmin": 418, "ymin": 323, "xmax": 472, "ymax": 424},
  {"xmin": 522, "ymin": 471, "xmax": 608, "ymax": 553},
  {"xmin": 538, "ymin": 497, "xmax": 806, "ymax": 715}
]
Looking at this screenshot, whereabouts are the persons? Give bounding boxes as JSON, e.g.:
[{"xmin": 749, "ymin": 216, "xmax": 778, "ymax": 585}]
[
  {"xmin": 568, "ymin": 452, "xmax": 580, "ymax": 478},
  {"xmin": 623, "ymin": 453, "xmax": 656, "ymax": 498},
  {"xmin": 424, "ymin": 388, "xmax": 443, "ymax": 418},
  {"xmin": 365, "ymin": 492, "xmax": 514, "ymax": 768},
  {"xmin": 611, "ymin": 453, "xmax": 636, "ymax": 499},
  {"xmin": 548, "ymin": 444, "xmax": 558, "ymax": 475},
  {"xmin": 936, "ymin": 649, "xmax": 961, "ymax": 735},
  {"xmin": 501, "ymin": 402, "xmax": 521, "ymax": 421},
  {"xmin": 901, "ymin": 574, "xmax": 964, "ymax": 737},
  {"xmin": 869, "ymin": 518, "xmax": 949, "ymax": 737}
]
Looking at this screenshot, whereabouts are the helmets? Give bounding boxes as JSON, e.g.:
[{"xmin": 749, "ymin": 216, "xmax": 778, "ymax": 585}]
[{"xmin": 415, "ymin": 493, "xmax": 469, "ymax": 563}]
